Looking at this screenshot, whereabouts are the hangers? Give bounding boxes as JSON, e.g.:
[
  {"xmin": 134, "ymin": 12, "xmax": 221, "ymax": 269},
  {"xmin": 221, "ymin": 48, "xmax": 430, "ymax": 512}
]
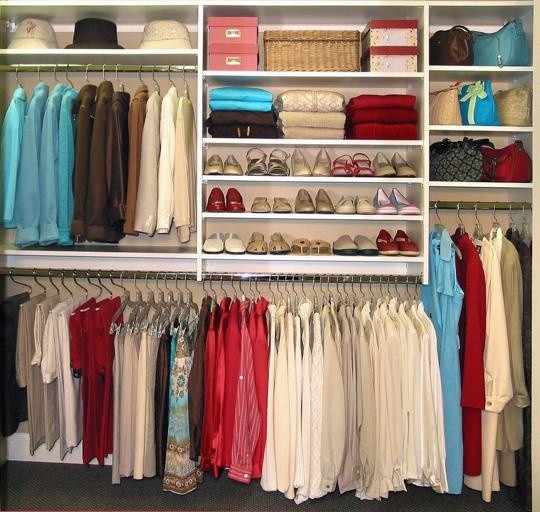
[
  {"xmin": 434, "ymin": 201, "xmax": 530, "ymax": 240},
  {"xmin": 15, "ymin": 62, "xmax": 189, "ymax": 98}
]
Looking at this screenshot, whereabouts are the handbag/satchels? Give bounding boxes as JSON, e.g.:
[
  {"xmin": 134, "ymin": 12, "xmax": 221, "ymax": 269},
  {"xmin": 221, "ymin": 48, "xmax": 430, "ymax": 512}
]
[
  {"xmin": 471, "ymin": 17, "xmax": 528, "ymax": 65},
  {"xmin": 429, "ymin": 25, "xmax": 474, "ymax": 65},
  {"xmin": 429, "ymin": 137, "xmax": 483, "ymax": 182},
  {"xmin": 493, "ymin": 83, "xmax": 532, "ymax": 126},
  {"xmin": 459, "ymin": 80, "xmax": 500, "ymax": 125},
  {"xmin": 429, "ymin": 85, "xmax": 462, "ymax": 124},
  {"xmin": 479, "ymin": 144, "xmax": 531, "ymax": 182}
]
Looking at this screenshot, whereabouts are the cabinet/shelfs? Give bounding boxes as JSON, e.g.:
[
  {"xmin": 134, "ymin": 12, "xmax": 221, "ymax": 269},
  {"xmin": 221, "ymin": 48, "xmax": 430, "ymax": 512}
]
[{"xmin": 0, "ymin": 0, "xmax": 540, "ymax": 469}]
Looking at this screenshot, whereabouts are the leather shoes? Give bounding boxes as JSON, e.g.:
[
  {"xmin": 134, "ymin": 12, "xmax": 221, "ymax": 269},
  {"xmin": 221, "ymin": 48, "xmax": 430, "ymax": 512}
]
[
  {"xmin": 203, "ymin": 230, "xmax": 419, "ymax": 255},
  {"xmin": 206, "ymin": 188, "xmax": 421, "ymax": 214},
  {"xmin": 203, "ymin": 146, "xmax": 417, "ymax": 176}
]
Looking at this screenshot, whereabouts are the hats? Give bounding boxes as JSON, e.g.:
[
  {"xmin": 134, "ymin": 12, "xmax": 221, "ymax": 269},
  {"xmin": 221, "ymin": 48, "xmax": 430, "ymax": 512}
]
[
  {"xmin": 7, "ymin": 18, "xmax": 59, "ymax": 49},
  {"xmin": 64, "ymin": 18, "xmax": 123, "ymax": 49},
  {"xmin": 138, "ymin": 20, "xmax": 192, "ymax": 49}
]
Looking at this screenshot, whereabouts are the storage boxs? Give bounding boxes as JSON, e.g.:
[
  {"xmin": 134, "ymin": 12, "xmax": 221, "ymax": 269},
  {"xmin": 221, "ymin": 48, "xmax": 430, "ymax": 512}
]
[
  {"xmin": 361, "ymin": 19, "xmax": 419, "ymax": 47},
  {"xmin": 206, "ymin": 43, "xmax": 260, "ymax": 71},
  {"xmin": 359, "ymin": 47, "xmax": 419, "ymax": 72},
  {"xmin": 208, "ymin": 16, "xmax": 259, "ymax": 43}
]
